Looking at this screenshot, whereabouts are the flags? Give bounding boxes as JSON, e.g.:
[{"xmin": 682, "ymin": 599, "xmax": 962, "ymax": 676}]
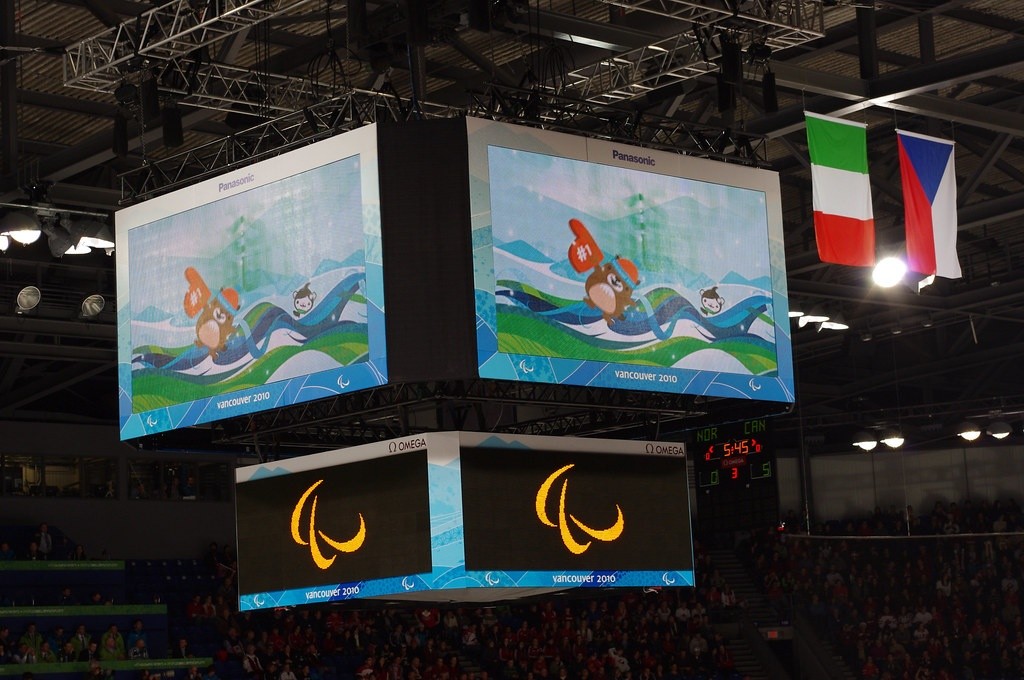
[
  {"xmin": 895, "ymin": 129, "xmax": 963, "ymax": 280},
  {"xmin": 803, "ymin": 110, "xmax": 877, "ymax": 267}
]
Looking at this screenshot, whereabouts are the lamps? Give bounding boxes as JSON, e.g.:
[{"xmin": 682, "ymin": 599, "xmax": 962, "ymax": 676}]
[
  {"xmin": 0, "ymin": 212, "xmax": 41, "ymax": 254},
  {"xmin": 44, "ymin": 220, "xmax": 115, "ymax": 257},
  {"xmin": 822, "ymin": 312, "xmax": 850, "ymax": 331},
  {"xmin": 853, "ymin": 430, "xmax": 877, "ymax": 452},
  {"xmin": 801, "ymin": 303, "xmax": 830, "ymax": 322},
  {"xmin": 921, "ymin": 319, "xmax": 933, "ymax": 328},
  {"xmin": 957, "ymin": 422, "xmax": 981, "ymax": 441},
  {"xmin": 72, "ymin": 293, "xmax": 105, "ymax": 322},
  {"xmin": 788, "ymin": 294, "xmax": 803, "ymax": 317},
  {"xmin": 691, "ymin": 23, "xmax": 745, "ymax": 90},
  {"xmin": 987, "ymin": 419, "xmax": 1012, "ymax": 440},
  {"xmin": 10, "ymin": 281, "xmax": 41, "ymax": 314},
  {"xmin": 861, "ymin": 332, "xmax": 872, "ymax": 342},
  {"xmin": 879, "ymin": 429, "xmax": 905, "ymax": 449},
  {"xmin": 133, "ymin": 49, "xmax": 164, "ymax": 124},
  {"xmin": 891, "ymin": 326, "xmax": 903, "ymax": 335}
]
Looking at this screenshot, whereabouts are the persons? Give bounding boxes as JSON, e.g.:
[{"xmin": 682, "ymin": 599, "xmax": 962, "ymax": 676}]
[{"xmin": 0, "ymin": 472, "xmax": 1024, "ymax": 679}]
